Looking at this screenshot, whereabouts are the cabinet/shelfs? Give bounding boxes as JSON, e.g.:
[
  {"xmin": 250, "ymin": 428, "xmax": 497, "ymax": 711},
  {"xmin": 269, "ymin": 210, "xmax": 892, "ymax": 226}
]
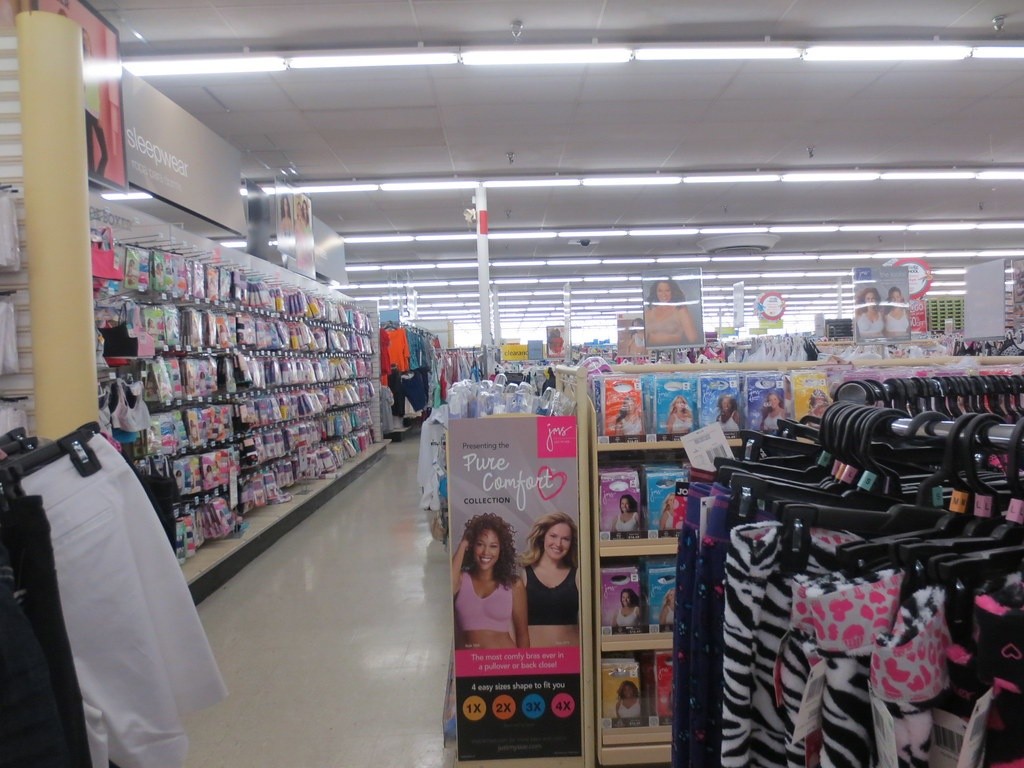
[{"xmin": 587, "ymin": 391, "xmax": 815, "ymax": 767}]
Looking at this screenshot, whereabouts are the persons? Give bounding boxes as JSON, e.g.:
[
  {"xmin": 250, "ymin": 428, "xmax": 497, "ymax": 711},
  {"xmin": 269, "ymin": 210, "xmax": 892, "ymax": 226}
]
[
  {"xmin": 660, "ymin": 492, "xmax": 675, "ymax": 529},
  {"xmin": 659, "ymin": 588, "xmax": 675, "ymax": 624},
  {"xmin": 453, "ymin": 512, "xmax": 530, "ymax": 649},
  {"xmin": 126, "ymin": 259, "xmax": 228, "ymax": 493},
  {"xmin": 516, "ymin": 512, "xmax": 578, "ymax": 647},
  {"xmin": 611, "ymin": 588, "xmax": 640, "ymax": 626},
  {"xmin": 611, "ymin": 494, "xmax": 639, "ymax": 532},
  {"xmin": 856, "ymin": 287, "xmax": 910, "ymax": 337},
  {"xmin": 616, "ymin": 680, "xmax": 640, "ymax": 717},
  {"xmin": 645, "ymin": 274, "xmax": 698, "ymax": 344},
  {"xmin": 628, "ymin": 318, "xmax": 648, "ymax": 355},
  {"xmin": 614, "ymin": 389, "xmax": 830, "ymax": 436},
  {"xmin": 280, "ymin": 195, "xmax": 311, "ymax": 236},
  {"xmin": 81, "ymin": 28, "xmax": 109, "ymax": 176}
]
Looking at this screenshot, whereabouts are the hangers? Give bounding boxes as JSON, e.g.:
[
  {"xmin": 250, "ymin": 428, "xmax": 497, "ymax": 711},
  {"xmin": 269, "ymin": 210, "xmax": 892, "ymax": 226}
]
[
  {"xmin": 0, "ymin": 420, "xmax": 106, "ymax": 545},
  {"xmin": 715, "ymin": 371, "xmax": 1024, "ymax": 658},
  {"xmin": 382, "ymin": 320, "xmax": 495, "ymax": 361},
  {"xmin": 608, "ymin": 323, "xmax": 1024, "ymax": 363}
]
